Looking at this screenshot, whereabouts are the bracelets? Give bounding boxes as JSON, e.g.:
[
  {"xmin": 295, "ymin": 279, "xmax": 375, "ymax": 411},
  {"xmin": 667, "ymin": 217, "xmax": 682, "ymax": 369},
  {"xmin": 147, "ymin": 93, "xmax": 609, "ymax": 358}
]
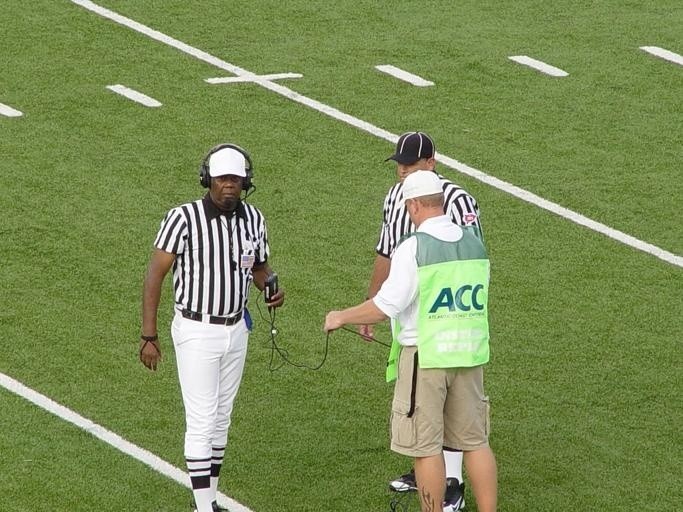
[{"xmin": 139, "ymin": 333, "xmax": 158, "ymax": 341}]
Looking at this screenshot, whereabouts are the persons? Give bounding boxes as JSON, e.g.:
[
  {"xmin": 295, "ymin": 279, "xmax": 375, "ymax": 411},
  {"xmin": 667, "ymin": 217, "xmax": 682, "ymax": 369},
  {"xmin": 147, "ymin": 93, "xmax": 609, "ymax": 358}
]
[
  {"xmin": 137, "ymin": 143, "xmax": 283, "ymax": 510},
  {"xmin": 359, "ymin": 129, "xmax": 481, "ymax": 511},
  {"xmin": 321, "ymin": 168, "xmax": 497, "ymax": 511}
]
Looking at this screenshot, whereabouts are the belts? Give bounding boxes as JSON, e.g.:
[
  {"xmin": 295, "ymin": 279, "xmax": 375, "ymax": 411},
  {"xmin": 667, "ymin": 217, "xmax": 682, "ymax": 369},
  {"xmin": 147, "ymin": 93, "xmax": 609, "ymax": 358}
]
[{"xmin": 181, "ymin": 309, "xmax": 244, "ymax": 326}]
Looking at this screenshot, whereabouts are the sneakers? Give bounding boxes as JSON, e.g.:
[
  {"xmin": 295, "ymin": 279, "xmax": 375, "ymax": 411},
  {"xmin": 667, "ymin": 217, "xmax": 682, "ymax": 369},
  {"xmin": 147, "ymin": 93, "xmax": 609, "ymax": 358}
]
[
  {"xmin": 443, "ymin": 477, "xmax": 467, "ymax": 512},
  {"xmin": 212, "ymin": 500, "xmax": 228, "ymax": 511},
  {"xmin": 389, "ymin": 471, "xmax": 421, "ymax": 492}
]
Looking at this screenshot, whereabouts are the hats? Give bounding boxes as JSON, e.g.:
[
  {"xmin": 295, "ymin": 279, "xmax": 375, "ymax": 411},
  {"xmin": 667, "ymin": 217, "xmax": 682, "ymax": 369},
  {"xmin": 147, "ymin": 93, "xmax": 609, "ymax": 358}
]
[
  {"xmin": 383, "ymin": 131, "xmax": 435, "ymax": 166},
  {"xmin": 394, "ymin": 170, "xmax": 444, "ymax": 210},
  {"xmin": 209, "ymin": 147, "xmax": 247, "ymax": 177}
]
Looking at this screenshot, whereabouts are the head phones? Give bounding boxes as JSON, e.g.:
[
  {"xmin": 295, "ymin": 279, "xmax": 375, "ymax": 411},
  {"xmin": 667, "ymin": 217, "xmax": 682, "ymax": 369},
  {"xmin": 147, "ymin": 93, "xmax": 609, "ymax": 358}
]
[{"xmin": 200, "ymin": 143, "xmax": 253, "ymax": 190}]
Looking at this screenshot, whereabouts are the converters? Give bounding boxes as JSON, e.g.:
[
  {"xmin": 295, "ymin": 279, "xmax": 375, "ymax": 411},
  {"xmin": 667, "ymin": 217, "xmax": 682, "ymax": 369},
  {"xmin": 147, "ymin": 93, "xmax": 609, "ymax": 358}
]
[{"xmin": 264, "ymin": 274, "xmax": 278, "ymax": 303}]
